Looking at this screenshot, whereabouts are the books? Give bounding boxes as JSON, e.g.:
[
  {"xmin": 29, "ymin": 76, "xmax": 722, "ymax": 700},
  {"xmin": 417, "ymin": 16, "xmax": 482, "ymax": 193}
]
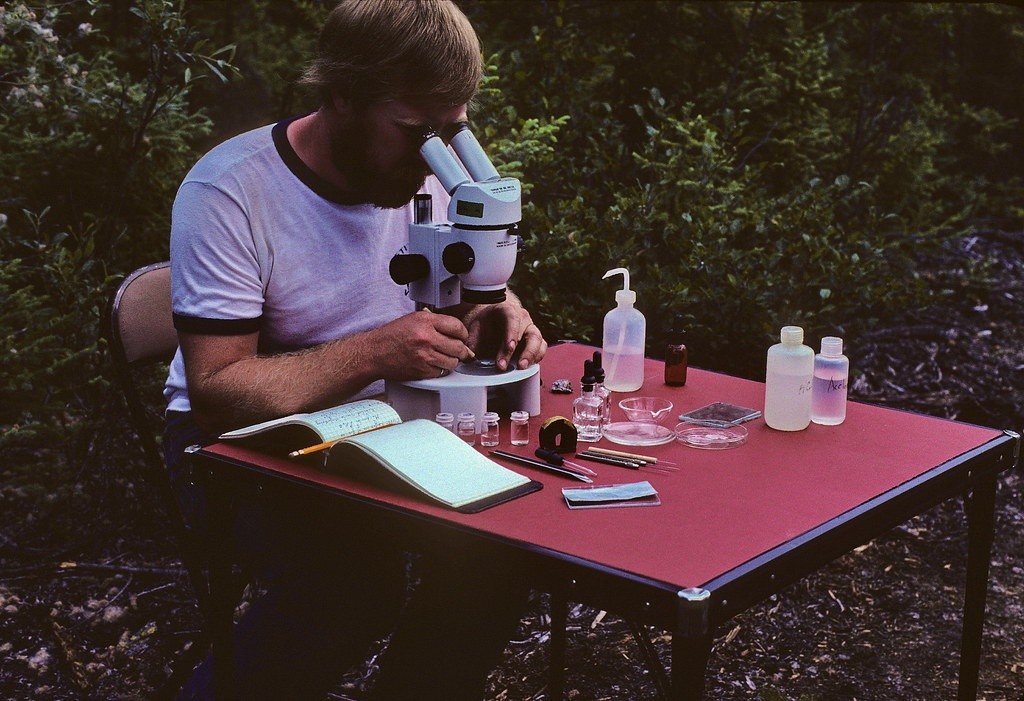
[
  {"xmin": 219, "ymin": 398, "xmax": 543, "ymax": 515},
  {"xmin": 560, "ymin": 481, "xmax": 662, "ymax": 509}
]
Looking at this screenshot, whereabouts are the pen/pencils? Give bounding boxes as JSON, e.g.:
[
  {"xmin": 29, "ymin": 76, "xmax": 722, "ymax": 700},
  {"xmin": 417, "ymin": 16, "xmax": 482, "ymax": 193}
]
[
  {"xmin": 574, "ymin": 446, "xmax": 658, "ymax": 471},
  {"xmin": 288, "ymin": 423, "xmax": 402, "ymax": 456}
]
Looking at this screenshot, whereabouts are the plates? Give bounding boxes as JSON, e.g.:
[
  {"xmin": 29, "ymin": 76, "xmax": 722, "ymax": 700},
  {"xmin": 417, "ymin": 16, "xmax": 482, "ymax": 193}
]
[
  {"xmin": 601, "ymin": 421, "xmax": 676, "ymax": 447},
  {"xmin": 675, "ymin": 420, "xmax": 749, "ymax": 450}
]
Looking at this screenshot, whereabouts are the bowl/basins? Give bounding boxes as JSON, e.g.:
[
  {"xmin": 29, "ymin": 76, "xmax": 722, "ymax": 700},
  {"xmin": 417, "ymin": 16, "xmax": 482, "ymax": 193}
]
[{"xmin": 618, "ymin": 395, "xmax": 674, "ymax": 423}]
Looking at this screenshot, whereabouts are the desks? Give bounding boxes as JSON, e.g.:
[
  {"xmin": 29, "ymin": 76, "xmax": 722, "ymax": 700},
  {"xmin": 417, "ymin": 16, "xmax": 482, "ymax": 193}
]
[{"xmin": 183, "ymin": 339, "xmax": 1021, "ymax": 701}]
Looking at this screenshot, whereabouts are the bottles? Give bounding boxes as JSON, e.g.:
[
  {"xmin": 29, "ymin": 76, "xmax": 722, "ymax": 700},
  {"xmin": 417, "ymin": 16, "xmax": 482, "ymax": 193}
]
[
  {"xmin": 663, "ymin": 314, "xmax": 690, "ymax": 387},
  {"xmin": 811, "ymin": 336, "xmax": 850, "ymax": 426},
  {"xmin": 763, "ymin": 325, "xmax": 815, "ymax": 432},
  {"xmin": 508, "ymin": 410, "xmax": 530, "ymax": 446},
  {"xmin": 600, "ymin": 290, "xmax": 646, "ymax": 394},
  {"xmin": 434, "ymin": 410, "xmax": 500, "ymax": 447},
  {"xmin": 570, "ymin": 351, "xmax": 612, "ymax": 442}
]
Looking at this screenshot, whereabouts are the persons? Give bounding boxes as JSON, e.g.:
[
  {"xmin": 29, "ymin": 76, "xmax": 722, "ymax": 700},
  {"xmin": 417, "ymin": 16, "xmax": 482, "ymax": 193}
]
[{"xmin": 164, "ymin": 0, "xmax": 547, "ymax": 701}]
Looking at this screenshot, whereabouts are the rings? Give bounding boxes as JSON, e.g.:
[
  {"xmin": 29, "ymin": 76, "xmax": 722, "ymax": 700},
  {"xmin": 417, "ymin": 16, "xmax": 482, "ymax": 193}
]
[{"xmin": 438, "ymin": 369, "xmax": 451, "ymax": 381}]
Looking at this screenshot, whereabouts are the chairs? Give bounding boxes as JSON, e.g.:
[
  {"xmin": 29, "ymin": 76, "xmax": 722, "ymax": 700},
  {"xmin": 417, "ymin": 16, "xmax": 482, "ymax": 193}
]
[{"xmin": 102, "ymin": 259, "xmax": 249, "ymax": 701}]
